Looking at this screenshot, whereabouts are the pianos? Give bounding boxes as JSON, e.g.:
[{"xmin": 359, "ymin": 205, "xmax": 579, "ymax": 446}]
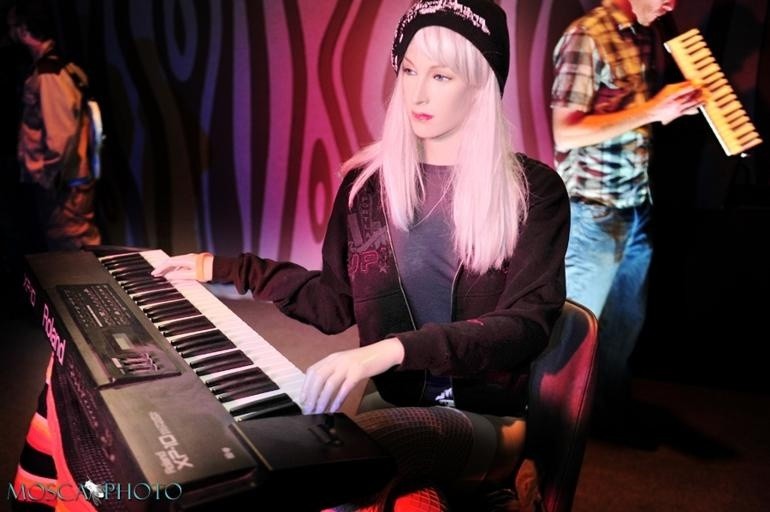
[{"xmin": 27, "ymin": 248, "xmax": 335, "ymax": 500}]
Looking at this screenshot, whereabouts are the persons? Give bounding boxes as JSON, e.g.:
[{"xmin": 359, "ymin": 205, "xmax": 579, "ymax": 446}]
[
  {"xmin": 549, "ymin": 0, "xmax": 707, "ymax": 452},
  {"xmin": 152, "ymin": 1, "xmax": 571, "ymax": 510},
  {"xmin": 9, "ymin": 5, "xmax": 104, "ymax": 253}
]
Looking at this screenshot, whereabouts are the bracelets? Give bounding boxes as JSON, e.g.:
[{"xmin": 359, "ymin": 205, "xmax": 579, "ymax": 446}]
[{"xmin": 197, "ymin": 251, "xmax": 211, "ymax": 282}]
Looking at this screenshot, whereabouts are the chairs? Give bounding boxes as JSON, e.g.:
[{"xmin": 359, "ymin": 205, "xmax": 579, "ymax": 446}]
[{"xmin": 382, "ymin": 296, "xmax": 602, "ymax": 512}]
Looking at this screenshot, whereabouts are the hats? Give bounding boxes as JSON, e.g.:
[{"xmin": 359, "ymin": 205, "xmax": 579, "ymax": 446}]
[{"xmin": 390, "ymin": 0, "xmax": 511, "ymax": 101}]
